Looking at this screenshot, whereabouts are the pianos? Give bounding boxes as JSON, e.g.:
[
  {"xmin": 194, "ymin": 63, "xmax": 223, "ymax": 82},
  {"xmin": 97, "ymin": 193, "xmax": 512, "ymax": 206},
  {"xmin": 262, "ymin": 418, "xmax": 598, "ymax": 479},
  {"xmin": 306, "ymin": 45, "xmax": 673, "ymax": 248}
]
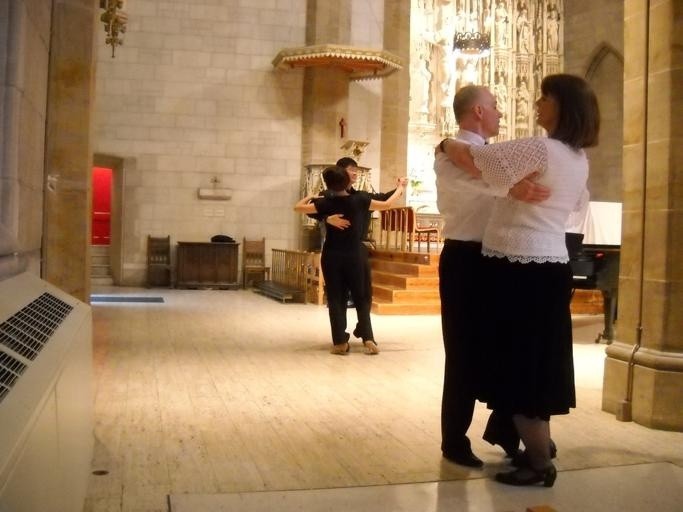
[{"xmin": 565, "ymin": 232, "xmax": 620, "ymax": 344}]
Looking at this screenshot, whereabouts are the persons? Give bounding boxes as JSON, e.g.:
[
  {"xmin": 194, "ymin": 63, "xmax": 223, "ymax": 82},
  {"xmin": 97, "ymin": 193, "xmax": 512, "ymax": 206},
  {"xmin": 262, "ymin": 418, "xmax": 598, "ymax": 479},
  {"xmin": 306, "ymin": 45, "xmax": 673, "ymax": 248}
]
[
  {"xmin": 295, "ymin": 166, "xmax": 406, "ymax": 354},
  {"xmin": 435, "ymin": 85, "xmax": 552, "ymax": 467},
  {"xmin": 306, "ymin": 158, "xmax": 407, "ymax": 352},
  {"xmin": 435, "ymin": 74, "xmax": 599, "ymax": 487}
]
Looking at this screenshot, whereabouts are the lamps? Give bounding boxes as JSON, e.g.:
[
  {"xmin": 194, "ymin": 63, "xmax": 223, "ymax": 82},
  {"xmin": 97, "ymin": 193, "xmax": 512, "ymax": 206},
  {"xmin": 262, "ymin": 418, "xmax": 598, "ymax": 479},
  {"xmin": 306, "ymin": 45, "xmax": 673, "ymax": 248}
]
[{"xmin": 450, "ymin": 0, "xmax": 493, "ymax": 57}]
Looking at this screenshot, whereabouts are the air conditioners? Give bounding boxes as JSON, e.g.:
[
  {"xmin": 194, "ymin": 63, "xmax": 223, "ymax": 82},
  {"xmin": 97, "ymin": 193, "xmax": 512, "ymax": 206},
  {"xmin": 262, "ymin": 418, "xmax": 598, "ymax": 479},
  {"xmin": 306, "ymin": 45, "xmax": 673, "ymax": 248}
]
[{"xmin": 0, "ymin": 268, "xmax": 93, "ymax": 511}]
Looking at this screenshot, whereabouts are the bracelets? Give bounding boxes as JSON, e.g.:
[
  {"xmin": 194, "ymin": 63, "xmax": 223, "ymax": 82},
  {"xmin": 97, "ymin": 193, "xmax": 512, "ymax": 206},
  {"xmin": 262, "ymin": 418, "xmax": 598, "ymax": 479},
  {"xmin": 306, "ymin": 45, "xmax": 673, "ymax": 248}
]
[{"xmin": 439, "ymin": 138, "xmax": 450, "ymax": 152}]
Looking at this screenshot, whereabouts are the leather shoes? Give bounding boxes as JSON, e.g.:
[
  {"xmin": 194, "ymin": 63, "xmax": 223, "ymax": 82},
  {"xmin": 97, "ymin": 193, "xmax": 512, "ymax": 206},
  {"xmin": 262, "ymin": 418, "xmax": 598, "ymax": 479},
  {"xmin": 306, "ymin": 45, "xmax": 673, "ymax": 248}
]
[
  {"xmin": 482, "ymin": 424, "xmax": 522, "ymax": 456},
  {"xmin": 442, "ymin": 449, "xmax": 483, "ymax": 467}
]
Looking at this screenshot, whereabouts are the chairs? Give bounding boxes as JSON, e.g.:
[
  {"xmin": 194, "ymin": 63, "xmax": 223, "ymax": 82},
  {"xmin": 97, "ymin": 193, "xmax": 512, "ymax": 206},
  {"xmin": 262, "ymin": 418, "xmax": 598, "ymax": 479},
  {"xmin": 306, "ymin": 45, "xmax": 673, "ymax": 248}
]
[
  {"xmin": 242, "ymin": 236, "xmax": 271, "ymax": 290},
  {"xmin": 145, "ymin": 234, "xmax": 170, "ymax": 289},
  {"xmin": 377, "ymin": 205, "xmax": 440, "ymax": 253}
]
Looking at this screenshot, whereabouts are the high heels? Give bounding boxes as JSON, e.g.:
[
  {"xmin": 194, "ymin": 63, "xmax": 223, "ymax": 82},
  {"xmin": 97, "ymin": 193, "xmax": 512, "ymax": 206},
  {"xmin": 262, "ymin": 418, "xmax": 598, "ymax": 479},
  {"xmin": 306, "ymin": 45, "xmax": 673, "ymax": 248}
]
[
  {"xmin": 497, "ymin": 463, "xmax": 556, "ymax": 487},
  {"xmin": 365, "ymin": 340, "xmax": 379, "ymax": 353},
  {"xmin": 511, "ymin": 440, "xmax": 556, "ymax": 465},
  {"xmin": 330, "ymin": 345, "xmax": 348, "ymax": 353}
]
